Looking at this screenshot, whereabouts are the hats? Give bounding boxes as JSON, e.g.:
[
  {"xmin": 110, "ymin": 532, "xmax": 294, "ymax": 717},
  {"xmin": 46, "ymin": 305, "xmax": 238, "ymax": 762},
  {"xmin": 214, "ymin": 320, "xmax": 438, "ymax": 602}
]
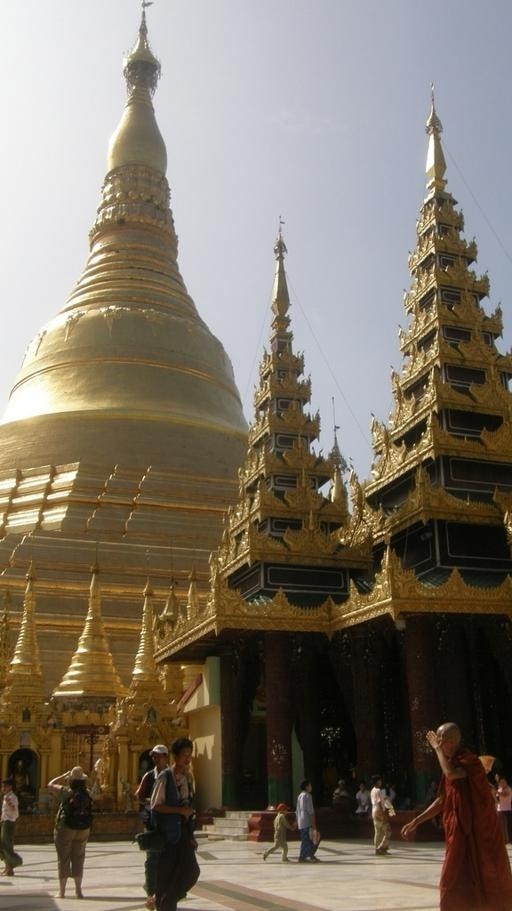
[
  {"xmin": 277, "ymin": 804, "xmax": 290, "ymax": 810},
  {"xmin": 149, "ymin": 745, "xmax": 168, "ymax": 756},
  {"xmin": 65, "ymin": 766, "xmax": 88, "ymax": 780}
]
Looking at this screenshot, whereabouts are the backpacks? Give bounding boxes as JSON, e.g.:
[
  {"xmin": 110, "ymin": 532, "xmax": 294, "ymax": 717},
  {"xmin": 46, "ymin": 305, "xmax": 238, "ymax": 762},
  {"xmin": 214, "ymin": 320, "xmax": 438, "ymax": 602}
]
[{"xmin": 65, "ymin": 789, "xmax": 92, "ymax": 830}]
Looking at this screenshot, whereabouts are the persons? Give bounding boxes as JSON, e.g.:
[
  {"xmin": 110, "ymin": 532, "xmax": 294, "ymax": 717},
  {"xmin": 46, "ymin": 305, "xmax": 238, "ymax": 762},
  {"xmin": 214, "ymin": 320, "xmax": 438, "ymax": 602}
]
[
  {"xmin": 0, "ymin": 780, "xmax": 22, "ymax": 878},
  {"xmin": 135, "ymin": 744, "xmax": 173, "ymax": 908},
  {"xmin": 400, "ymin": 723, "xmax": 512, "ymax": 910},
  {"xmin": 261, "ymin": 768, "xmax": 512, "ymax": 864},
  {"xmin": 150, "ymin": 741, "xmax": 201, "ymax": 911},
  {"xmin": 49, "ymin": 765, "xmax": 93, "ymax": 898}
]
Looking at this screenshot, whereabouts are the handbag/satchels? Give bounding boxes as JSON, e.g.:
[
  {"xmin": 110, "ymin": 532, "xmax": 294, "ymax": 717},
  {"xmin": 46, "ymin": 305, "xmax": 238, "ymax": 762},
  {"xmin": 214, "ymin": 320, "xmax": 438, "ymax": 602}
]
[{"xmin": 136, "ymin": 831, "xmax": 165, "ymax": 850}]
